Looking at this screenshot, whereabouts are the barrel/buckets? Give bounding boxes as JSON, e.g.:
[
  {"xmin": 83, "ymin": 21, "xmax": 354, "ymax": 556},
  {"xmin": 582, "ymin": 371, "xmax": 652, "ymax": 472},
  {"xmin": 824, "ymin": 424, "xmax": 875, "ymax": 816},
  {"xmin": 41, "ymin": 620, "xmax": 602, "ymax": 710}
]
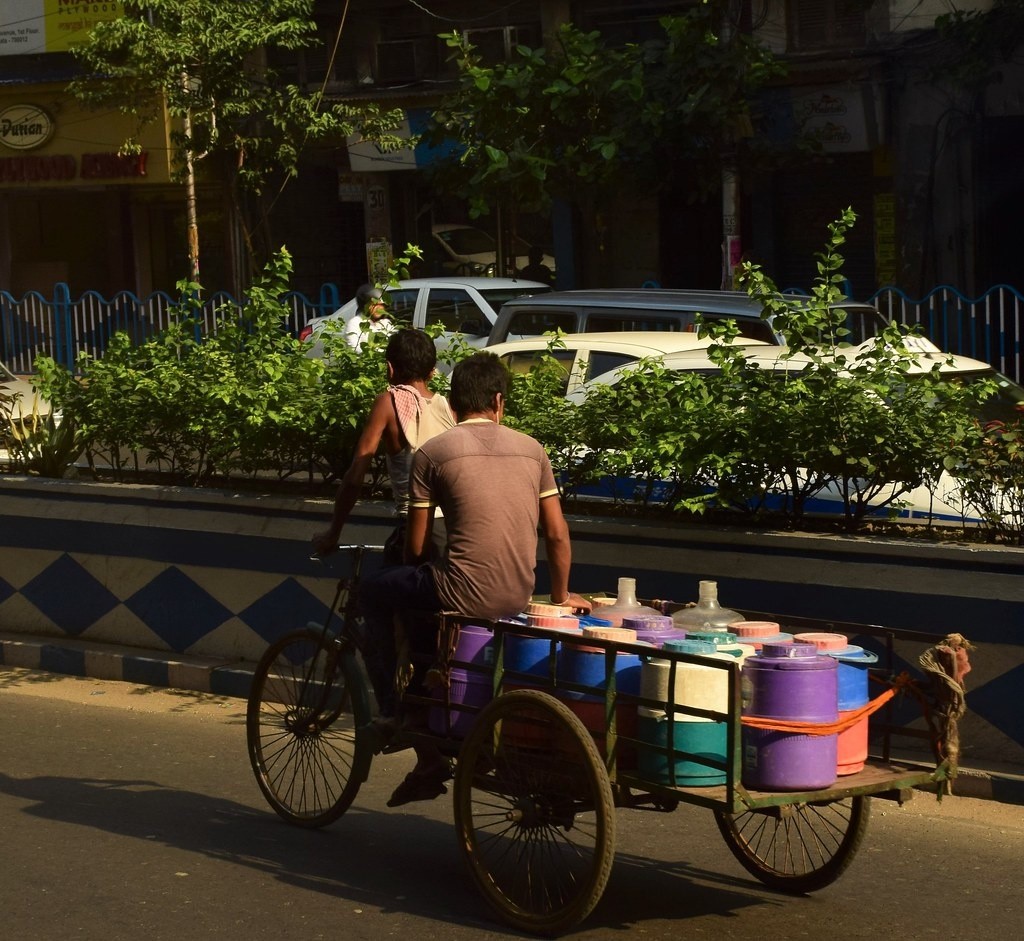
[{"xmin": 434, "ymin": 598, "xmax": 877, "ymax": 791}]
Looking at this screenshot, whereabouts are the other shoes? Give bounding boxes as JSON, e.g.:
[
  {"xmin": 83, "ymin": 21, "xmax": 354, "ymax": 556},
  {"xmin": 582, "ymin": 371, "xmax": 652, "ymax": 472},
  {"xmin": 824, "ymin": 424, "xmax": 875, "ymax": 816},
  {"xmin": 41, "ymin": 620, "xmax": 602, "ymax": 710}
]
[
  {"xmin": 386, "ymin": 762, "xmax": 453, "ymax": 807},
  {"xmin": 357, "ymin": 717, "xmax": 417, "ymax": 752}
]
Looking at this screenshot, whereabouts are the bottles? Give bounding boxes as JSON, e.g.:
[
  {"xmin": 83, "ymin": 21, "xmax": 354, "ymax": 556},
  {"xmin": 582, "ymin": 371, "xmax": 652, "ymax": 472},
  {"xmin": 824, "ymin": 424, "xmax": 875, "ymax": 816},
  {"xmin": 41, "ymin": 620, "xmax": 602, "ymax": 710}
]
[
  {"xmin": 671, "ymin": 581, "xmax": 746, "ymax": 632},
  {"xmin": 591, "ymin": 577, "xmax": 664, "ymax": 628}
]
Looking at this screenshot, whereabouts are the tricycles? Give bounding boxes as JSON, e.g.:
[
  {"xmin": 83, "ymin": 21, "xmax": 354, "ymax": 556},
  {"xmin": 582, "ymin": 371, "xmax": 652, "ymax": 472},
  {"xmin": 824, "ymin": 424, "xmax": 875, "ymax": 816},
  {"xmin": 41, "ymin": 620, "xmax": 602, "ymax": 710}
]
[{"xmin": 246, "ymin": 527, "xmax": 967, "ymax": 941}]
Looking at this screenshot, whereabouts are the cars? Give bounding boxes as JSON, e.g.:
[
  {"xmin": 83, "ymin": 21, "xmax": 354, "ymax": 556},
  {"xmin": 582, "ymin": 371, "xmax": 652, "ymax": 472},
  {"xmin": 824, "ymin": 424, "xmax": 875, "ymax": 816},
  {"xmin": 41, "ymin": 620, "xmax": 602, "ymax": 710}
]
[
  {"xmin": 549, "ymin": 334, "xmax": 1024, "ymax": 524},
  {"xmin": 0, "ymin": 363, "xmax": 64, "ymax": 465},
  {"xmin": 303, "ymin": 277, "xmax": 553, "ymax": 392},
  {"xmin": 437, "ymin": 332, "xmax": 772, "ymax": 402}
]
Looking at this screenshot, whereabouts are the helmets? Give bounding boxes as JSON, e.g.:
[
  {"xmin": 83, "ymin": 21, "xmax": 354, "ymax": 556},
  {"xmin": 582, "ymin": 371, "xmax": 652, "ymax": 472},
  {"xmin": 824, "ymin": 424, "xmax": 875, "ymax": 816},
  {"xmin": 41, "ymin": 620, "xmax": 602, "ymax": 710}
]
[{"xmin": 368, "ymin": 287, "xmax": 388, "ymax": 305}]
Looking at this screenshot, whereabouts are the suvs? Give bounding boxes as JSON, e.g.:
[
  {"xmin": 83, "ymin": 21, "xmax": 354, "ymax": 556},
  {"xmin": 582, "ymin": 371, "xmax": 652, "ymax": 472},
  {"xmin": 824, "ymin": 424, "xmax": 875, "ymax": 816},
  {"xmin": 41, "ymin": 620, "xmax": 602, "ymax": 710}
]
[{"xmin": 486, "ymin": 286, "xmax": 906, "ymax": 363}]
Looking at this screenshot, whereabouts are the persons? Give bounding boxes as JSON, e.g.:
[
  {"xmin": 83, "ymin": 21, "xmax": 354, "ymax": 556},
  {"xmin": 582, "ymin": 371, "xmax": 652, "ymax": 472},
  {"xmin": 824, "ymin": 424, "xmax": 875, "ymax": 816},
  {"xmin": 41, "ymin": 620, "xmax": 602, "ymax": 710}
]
[
  {"xmin": 361, "ymin": 353, "xmax": 571, "ymax": 718},
  {"xmin": 340, "ymin": 285, "xmax": 398, "ymax": 352},
  {"xmin": 311, "ymin": 329, "xmax": 448, "ymax": 808}
]
